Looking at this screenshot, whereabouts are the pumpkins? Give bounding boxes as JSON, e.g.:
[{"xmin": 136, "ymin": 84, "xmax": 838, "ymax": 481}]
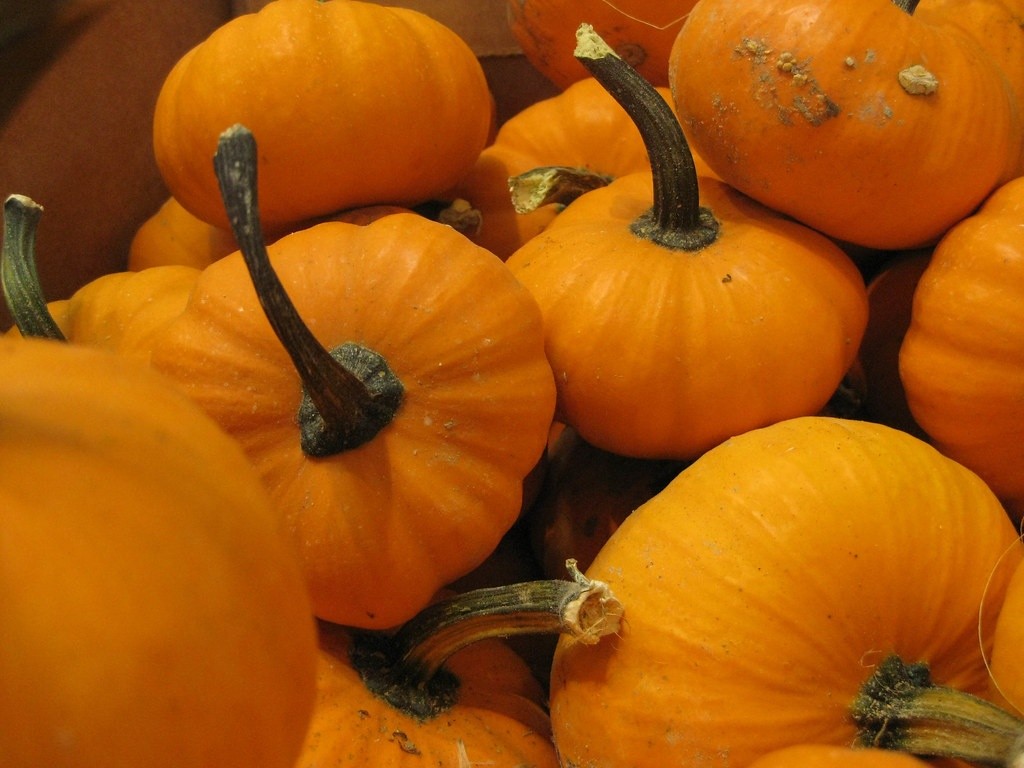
[{"xmin": 0, "ymin": 0, "xmax": 1024, "ymax": 768}]
